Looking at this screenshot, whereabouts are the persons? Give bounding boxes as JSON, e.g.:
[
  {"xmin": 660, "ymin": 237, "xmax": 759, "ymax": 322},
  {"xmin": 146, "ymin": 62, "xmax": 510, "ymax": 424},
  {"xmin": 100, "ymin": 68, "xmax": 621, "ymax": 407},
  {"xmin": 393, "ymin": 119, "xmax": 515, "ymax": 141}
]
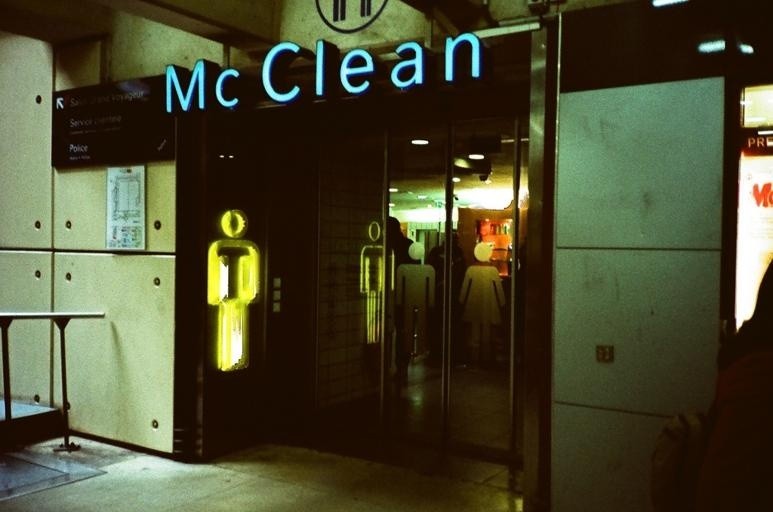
[
  {"xmin": 426, "ymin": 231, "xmax": 465, "ymax": 365},
  {"xmin": 386, "ymin": 217, "xmax": 422, "ymax": 384}
]
